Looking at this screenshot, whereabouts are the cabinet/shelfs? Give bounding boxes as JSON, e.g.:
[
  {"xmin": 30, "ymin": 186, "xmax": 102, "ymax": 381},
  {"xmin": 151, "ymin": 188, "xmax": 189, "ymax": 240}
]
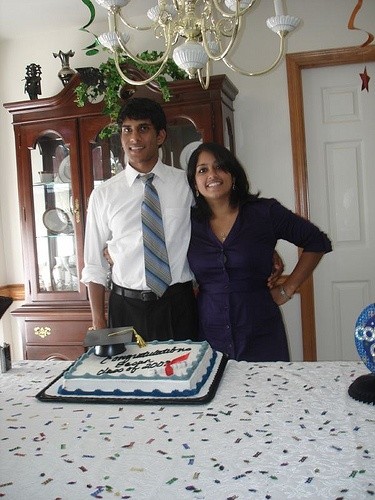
[{"xmin": 2, "ymin": 60, "xmax": 241, "ymax": 361}]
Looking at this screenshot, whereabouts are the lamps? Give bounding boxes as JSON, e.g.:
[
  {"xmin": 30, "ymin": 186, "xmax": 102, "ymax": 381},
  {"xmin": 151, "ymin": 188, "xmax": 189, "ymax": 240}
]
[{"xmin": 96, "ymin": 0, "xmax": 299, "ymax": 90}]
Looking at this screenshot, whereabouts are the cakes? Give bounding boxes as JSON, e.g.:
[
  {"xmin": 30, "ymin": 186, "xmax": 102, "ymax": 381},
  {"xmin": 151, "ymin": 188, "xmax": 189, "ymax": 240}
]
[{"xmin": 57, "ymin": 326, "xmax": 217, "ymax": 397}]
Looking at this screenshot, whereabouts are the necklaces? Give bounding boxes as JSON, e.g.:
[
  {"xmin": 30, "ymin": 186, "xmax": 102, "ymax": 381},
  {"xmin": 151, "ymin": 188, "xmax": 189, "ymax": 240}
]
[{"xmin": 211, "ymin": 209, "xmax": 237, "ymax": 239}]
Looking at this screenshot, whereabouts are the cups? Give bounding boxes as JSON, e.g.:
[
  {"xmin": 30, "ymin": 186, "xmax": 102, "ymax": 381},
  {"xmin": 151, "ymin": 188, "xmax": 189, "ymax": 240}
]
[
  {"xmin": 0, "ymin": 344, "xmax": 12, "ymax": 372},
  {"xmin": 37, "ymin": 171, "xmax": 57, "ymax": 183}
]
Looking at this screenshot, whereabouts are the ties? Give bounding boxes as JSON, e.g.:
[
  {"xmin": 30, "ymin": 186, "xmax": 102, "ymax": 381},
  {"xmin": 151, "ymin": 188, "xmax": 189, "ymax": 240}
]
[{"xmin": 137, "ymin": 173, "xmax": 172, "ymax": 298}]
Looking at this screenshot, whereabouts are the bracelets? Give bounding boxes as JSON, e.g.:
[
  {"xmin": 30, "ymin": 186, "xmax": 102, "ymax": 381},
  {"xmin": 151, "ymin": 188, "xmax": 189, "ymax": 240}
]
[{"xmin": 280, "ymin": 284, "xmax": 292, "ymax": 300}]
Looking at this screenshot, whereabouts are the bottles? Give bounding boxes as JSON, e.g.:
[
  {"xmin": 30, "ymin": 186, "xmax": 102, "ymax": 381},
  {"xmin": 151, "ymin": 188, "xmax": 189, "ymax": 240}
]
[{"xmin": 53, "ymin": 257, "xmax": 71, "ymax": 292}]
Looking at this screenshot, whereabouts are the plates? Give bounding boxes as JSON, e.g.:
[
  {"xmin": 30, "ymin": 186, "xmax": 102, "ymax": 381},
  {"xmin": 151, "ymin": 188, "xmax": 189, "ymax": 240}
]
[
  {"xmin": 179, "ymin": 140, "xmax": 205, "ymax": 169},
  {"xmin": 43, "ymin": 208, "xmax": 69, "ymax": 232},
  {"xmin": 59, "ymin": 154, "xmax": 72, "ymax": 183}
]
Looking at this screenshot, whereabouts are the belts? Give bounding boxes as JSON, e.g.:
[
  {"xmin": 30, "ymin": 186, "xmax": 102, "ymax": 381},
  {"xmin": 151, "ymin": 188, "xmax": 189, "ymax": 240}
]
[{"xmin": 110, "ymin": 280, "xmax": 194, "ymax": 300}]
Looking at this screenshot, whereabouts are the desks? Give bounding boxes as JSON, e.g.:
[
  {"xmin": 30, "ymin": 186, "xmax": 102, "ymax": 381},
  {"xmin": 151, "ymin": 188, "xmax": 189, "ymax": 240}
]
[{"xmin": 0, "ymin": 361, "xmax": 375, "ymax": 500}]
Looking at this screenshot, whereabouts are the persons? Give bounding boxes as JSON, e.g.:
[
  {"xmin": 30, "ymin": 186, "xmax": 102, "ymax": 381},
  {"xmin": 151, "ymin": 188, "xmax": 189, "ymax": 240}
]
[
  {"xmin": 187, "ymin": 143, "xmax": 332, "ymax": 362},
  {"xmin": 80, "ymin": 97, "xmax": 284, "ymax": 342}
]
